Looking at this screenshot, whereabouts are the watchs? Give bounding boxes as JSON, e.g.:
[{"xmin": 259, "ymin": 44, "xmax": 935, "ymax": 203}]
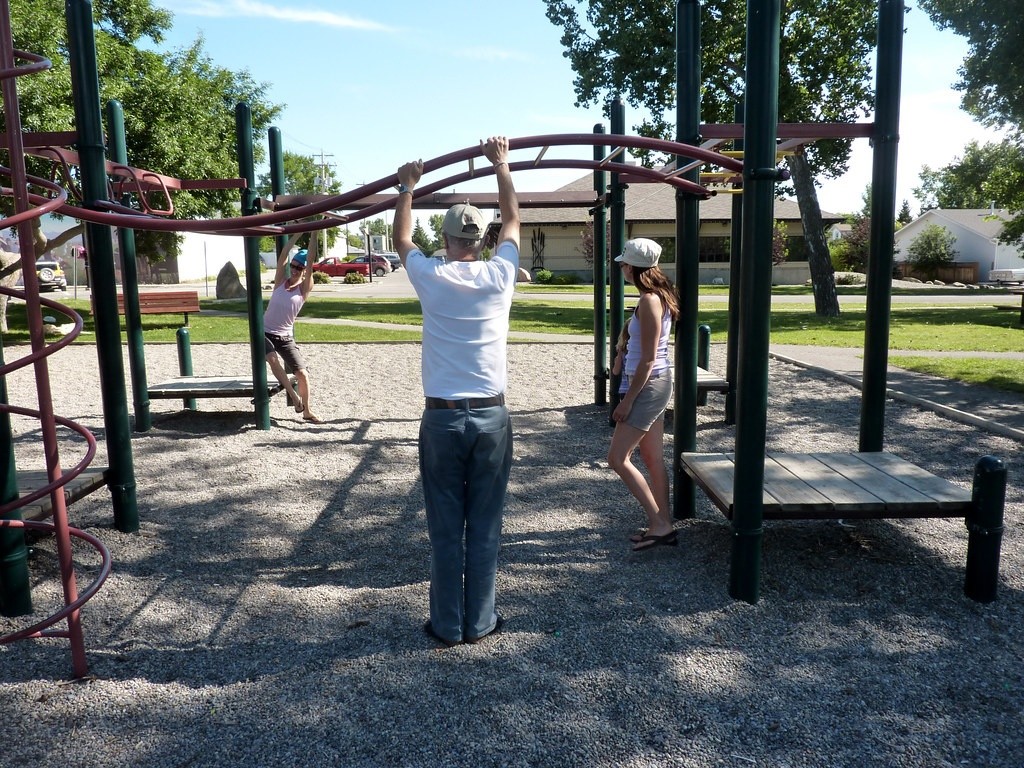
[{"xmin": 399, "ymin": 184, "xmax": 413, "ymax": 195}]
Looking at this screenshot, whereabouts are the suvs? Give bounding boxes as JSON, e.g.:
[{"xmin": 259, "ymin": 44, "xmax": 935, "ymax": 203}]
[
  {"xmin": 377, "ymin": 253, "xmax": 404, "ymax": 272},
  {"xmin": 34, "ymin": 261, "xmax": 68, "ymax": 293}
]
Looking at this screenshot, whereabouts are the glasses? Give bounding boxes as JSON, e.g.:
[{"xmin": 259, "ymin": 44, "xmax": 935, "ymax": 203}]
[
  {"xmin": 618, "ymin": 264, "xmax": 627, "ymax": 270},
  {"xmin": 290, "ymin": 263, "xmax": 306, "ymax": 271}
]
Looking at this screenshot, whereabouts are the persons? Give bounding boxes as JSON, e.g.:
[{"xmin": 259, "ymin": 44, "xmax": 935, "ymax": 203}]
[
  {"xmin": 264, "ymin": 220, "xmax": 327, "ymax": 424},
  {"xmin": 393, "ymin": 137, "xmax": 520, "ymax": 646},
  {"xmin": 31, "ymin": 217, "xmax": 47, "ymax": 250},
  {"xmin": 607, "ymin": 238, "xmax": 680, "ymax": 550}
]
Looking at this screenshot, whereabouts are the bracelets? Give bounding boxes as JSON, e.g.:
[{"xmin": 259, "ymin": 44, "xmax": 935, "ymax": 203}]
[{"xmin": 494, "ymin": 161, "xmax": 509, "ymax": 171}]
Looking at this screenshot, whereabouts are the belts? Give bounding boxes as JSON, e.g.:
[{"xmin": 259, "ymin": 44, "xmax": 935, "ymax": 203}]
[{"xmin": 426, "ymin": 393, "xmax": 505, "ymax": 410}]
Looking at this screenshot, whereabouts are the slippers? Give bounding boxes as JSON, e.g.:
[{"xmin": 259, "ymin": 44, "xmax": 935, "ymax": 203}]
[
  {"xmin": 304, "ymin": 415, "xmax": 322, "ymax": 424},
  {"xmin": 295, "ymin": 399, "xmax": 305, "ymax": 413},
  {"xmin": 634, "ymin": 531, "xmax": 678, "ymax": 552},
  {"xmin": 631, "ymin": 533, "xmax": 679, "ymax": 546}
]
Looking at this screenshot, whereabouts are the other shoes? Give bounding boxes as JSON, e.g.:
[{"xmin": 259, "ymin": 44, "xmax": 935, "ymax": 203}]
[
  {"xmin": 465, "ymin": 615, "xmax": 503, "ymax": 644},
  {"xmin": 425, "ymin": 619, "xmax": 463, "ymax": 647}
]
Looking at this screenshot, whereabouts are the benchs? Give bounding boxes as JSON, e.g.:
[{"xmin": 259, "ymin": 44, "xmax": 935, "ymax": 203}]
[{"xmin": 88, "ymin": 292, "xmax": 201, "ymax": 326}]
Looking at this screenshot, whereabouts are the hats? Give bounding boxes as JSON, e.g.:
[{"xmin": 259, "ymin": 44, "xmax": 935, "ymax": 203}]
[
  {"xmin": 614, "ymin": 238, "xmax": 662, "ymax": 268},
  {"xmin": 293, "ymin": 249, "xmax": 309, "ymax": 267},
  {"xmin": 442, "ymin": 204, "xmax": 485, "ymax": 240}
]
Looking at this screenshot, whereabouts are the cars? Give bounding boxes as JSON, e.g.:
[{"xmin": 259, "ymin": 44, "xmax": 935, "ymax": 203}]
[{"xmin": 347, "ymin": 254, "xmax": 392, "ymax": 277}]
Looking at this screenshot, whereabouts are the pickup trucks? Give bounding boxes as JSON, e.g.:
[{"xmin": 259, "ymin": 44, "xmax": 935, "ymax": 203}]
[{"xmin": 312, "ymin": 256, "xmax": 370, "ymax": 278}]
